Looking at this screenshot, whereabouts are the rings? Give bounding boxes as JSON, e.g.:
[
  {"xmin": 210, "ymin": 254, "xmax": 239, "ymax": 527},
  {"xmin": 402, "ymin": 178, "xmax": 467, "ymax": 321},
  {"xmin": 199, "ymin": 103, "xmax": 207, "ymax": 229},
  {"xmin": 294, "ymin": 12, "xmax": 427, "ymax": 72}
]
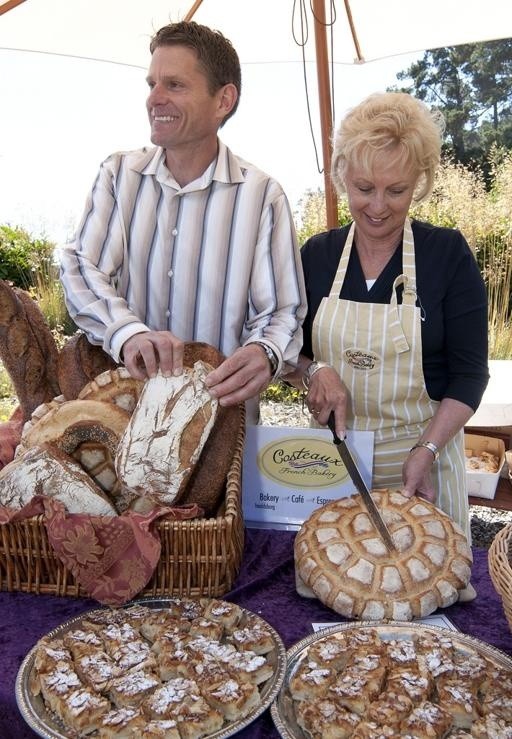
[{"xmin": 312, "ymin": 408, "xmax": 320, "ymax": 416}]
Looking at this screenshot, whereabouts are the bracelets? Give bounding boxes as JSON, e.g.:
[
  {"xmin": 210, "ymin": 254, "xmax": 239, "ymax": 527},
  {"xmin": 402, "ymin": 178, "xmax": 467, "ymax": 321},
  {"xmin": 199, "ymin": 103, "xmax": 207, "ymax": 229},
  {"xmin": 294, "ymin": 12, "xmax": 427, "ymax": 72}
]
[
  {"xmin": 300, "ymin": 360, "xmax": 335, "ymax": 389},
  {"xmin": 410, "ymin": 440, "xmax": 441, "ymax": 462}
]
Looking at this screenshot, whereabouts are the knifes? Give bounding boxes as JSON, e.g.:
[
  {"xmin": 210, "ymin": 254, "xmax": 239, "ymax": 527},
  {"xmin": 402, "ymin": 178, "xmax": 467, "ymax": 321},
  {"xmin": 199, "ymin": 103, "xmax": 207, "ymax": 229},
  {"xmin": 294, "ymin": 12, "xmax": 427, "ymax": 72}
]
[{"xmin": 325, "ymin": 411, "xmax": 397, "ymax": 554}]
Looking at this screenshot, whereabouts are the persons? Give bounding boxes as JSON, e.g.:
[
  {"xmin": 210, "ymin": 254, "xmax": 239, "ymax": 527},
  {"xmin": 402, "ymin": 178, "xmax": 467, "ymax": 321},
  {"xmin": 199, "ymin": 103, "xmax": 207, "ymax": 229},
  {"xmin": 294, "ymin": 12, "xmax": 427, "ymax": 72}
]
[
  {"xmin": 55, "ymin": 20, "xmax": 310, "ymax": 427},
  {"xmin": 277, "ymin": 88, "xmax": 489, "ymax": 544}
]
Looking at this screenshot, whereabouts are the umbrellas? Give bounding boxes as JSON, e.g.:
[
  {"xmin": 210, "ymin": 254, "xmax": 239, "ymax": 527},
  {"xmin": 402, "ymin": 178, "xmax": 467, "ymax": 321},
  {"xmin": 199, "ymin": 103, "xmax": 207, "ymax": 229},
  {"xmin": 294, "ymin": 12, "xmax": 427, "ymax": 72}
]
[{"xmin": 0, "ymin": 0, "xmax": 511, "ymax": 230}]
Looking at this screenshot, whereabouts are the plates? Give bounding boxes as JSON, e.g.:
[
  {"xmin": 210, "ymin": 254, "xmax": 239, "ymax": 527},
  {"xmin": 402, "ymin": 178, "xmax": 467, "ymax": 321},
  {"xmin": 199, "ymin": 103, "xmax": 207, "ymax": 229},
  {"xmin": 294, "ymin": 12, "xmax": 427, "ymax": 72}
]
[{"xmin": 269, "ymin": 618, "xmax": 511, "ymax": 739}]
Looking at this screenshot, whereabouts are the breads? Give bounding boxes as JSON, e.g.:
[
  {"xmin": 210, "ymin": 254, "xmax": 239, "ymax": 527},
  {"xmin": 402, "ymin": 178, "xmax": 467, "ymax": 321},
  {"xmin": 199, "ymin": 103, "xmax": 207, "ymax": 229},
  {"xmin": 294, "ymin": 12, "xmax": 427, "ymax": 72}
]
[
  {"xmin": 288, "ymin": 625, "xmax": 512, "ymax": 738},
  {"xmin": 288, "ymin": 627, "xmax": 512, "ymax": 739},
  {"xmin": 0, "ymin": 279, "xmax": 240, "ymax": 517},
  {"xmin": 31, "ymin": 597, "xmax": 275, "ymax": 738}
]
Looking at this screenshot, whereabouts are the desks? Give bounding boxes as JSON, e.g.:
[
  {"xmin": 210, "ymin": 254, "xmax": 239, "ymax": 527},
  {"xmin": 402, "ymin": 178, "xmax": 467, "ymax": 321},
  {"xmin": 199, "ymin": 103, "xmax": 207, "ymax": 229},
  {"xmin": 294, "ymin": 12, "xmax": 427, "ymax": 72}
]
[{"xmin": 1, "ymin": 527, "xmax": 512, "ymax": 739}]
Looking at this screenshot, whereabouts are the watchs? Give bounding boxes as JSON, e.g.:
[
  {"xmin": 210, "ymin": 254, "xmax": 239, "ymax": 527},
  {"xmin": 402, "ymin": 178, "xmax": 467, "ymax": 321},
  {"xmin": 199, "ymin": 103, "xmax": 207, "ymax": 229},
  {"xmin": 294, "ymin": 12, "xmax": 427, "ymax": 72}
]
[{"xmin": 257, "ymin": 340, "xmax": 280, "ymax": 374}]
